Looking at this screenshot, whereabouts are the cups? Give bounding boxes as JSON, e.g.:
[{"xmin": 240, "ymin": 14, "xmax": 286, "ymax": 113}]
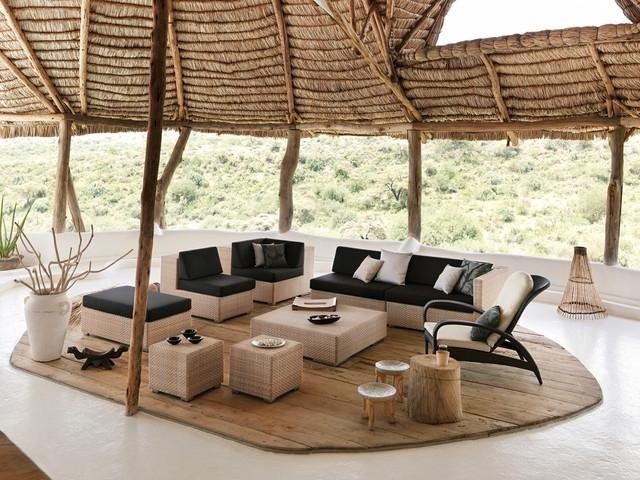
[
  {"xmin": 438, "ymin": 345, "xmax": 447, "ymax": 353},
  {"xmin": 436, "ymin": 351, "xmax": 452, "ymax": 366}
]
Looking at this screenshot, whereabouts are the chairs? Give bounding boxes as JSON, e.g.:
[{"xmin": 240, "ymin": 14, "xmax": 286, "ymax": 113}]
[
  {"xmin": 422, "ymin": 269, "xmax": 550, "ymax": 388},
  {"xmin": 217, "ymin": 236, "xmax": 316, "ymax": 305},
  {"xmin": 161, "ymin": 247, "xmax": 257, "ymax": 323}
]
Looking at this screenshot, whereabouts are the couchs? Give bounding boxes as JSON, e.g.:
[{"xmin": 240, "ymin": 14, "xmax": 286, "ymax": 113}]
[{"xmin": 308, "ymin": 245, "xmax": 509, "ymax": 335}]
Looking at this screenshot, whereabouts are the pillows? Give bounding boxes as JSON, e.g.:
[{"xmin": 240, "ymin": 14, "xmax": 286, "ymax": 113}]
[
  {"xmin": 251, "ymin": 241, "xmax": 276, "ymax": 267},
  {"xmin": 457, "ymin": 258, "xmax": 494, "ymax": 298},
  {"xmin": 376, "ymin": 247, "xmax": 414, "ymax": 288},
  {"xmin": 432, "ymin": 264, "xmax": 465, "ymax": 295},
  {"xmin": 352, "ymin": 254, "xmax": 385, "ymax": 284},
  {"xmin": 261, "ymin": 242, "xmax": 290, "ymax": 270},
  {"xmin": 469, "ymin": 304, "xmax": 502, "ymax": 340}
]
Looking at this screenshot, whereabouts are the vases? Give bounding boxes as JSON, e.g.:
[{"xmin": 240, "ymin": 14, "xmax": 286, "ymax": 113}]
[{"xmin": 25, "ymin": 288, "xmax": 73, "ymax": 363}]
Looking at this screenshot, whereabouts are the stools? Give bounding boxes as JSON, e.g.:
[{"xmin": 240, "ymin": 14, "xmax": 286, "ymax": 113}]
[
  {"xmin": 230, "ymin": 334, "xmax": 303, "ymax": 403},
  {"xmin": 148, "ymin": 334, "xmax": 223, "ymax": 401},
  {"xmin": 373, "ymin": 358, "xmax": 409, "ymax": 403},
  {"xmin": 355, "ymin": 382, "xmax": 399, "ymax": 432}
]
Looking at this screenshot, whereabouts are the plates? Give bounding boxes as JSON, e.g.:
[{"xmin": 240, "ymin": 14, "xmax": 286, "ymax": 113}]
[
  {"xmin": 251, "ymin": 339, "xmax": 286, "ymax": 348},
  {"xmin": 308, "ymin": 315, "xmax": 340, "ymax": 324}
]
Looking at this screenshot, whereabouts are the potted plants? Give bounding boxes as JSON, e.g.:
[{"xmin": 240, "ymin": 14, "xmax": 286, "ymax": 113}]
[{"xmin": 0, "ymin": 192, "xmax": 39, "ymax": 270}]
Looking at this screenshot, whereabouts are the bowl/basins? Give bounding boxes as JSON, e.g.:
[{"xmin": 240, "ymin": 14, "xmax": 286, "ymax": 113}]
[
  {"xmin": 187, "ymin": 337, "xmax": 204, "ymax": 343},
  {"xmin": 166, "ymin": 337, "xmax": 181, "ymax": 346},
  {"xmin": 180, "ymin": 328, "xmax": 197, "ymax": 338}
]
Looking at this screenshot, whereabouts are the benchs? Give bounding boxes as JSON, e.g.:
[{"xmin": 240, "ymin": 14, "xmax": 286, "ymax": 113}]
[{"xmin": 80, "ymin": 285, "xmax": 193, "ymax": 351}]
